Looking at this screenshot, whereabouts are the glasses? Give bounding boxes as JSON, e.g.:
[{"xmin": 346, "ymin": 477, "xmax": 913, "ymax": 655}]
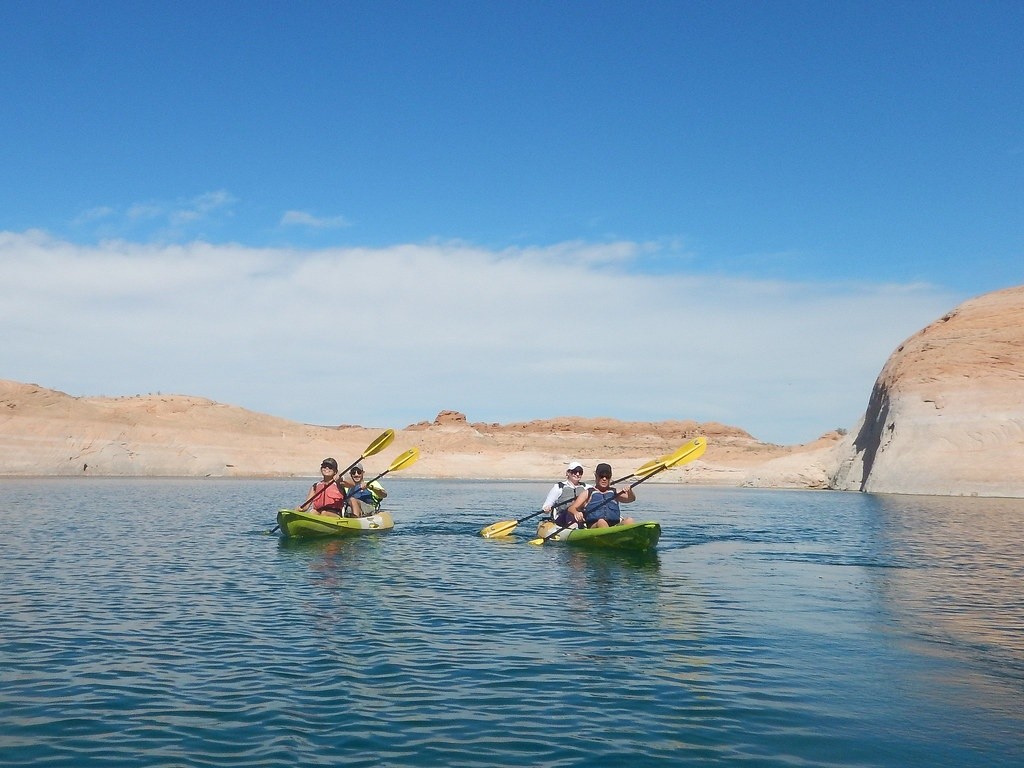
[
  {"xmin": 322, "ymin": 464, "xmax": 333, "ymax": 469},
  {"xmin": 351, "ymin": 470, "xmax": 362, "ymax": 475},
  {"xmin": 598, "ymin": 474, "xmax": 610, "ymax": 479},
  {"xmin": 571, "ymin": 470, "xmax": 583, "ymax": 475}
]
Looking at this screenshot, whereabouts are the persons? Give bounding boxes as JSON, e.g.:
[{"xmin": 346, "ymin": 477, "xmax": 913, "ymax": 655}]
[
  {"xmin": 541, "ymin": 462, "xmax": 593, "ymax": 528},
  {"xmin": 345, "ymin": 463, "xmax": 388, "ymax": 518},
  {"xmin": 295, "ymin": 458, "xmax": 355, "ymax": 518},
  {"xmin": 567, "ymin": 464, "xmax": 636, "ymax": 529}
]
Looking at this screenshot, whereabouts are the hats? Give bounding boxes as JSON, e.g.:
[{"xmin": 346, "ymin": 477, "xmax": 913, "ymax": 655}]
[
  {"xmin": 350, "ymin": 463, "xmax": 363, "ymax": 471},
  {"xmin": 321, "ymin": 458, "xmax": 337, "ymax": 469},
  {"xmin": 596, "ymin": 464, "xmax": 612, "ymax": 476},
  {"xmin": 567, "ymin": 462, "xmax": 584, "ymax": 471}
]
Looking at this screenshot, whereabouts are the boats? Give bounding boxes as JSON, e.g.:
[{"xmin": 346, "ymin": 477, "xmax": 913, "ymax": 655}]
[
  {"xmin": 536, "ymin": 520, "xmax": 665, "ymax": 552},
  {"xmin": 273, "ymin": 508, "xmax": 396, "ymax": 541}
]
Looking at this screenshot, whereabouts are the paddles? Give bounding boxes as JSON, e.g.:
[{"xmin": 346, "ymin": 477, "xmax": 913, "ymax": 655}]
[
  {"xmin": 268, "ymin": 427, "xmax": 395, "ymax": 537},
  {"xmin": 315, "ymin": 446, "xmax": 422, "ymax": 513},
  {"xmin": 476, "ymin": 436, "xmax": 709, "ymax": 547}
]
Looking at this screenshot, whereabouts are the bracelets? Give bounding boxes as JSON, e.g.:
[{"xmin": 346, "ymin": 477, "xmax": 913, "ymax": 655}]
[{"xmin": 369, "ymin": 485, "xmax": 373, "ymax": 490}]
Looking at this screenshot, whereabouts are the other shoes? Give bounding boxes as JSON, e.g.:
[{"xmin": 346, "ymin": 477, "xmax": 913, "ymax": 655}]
[{"xmin": 344, "ymin": 512, "xmax": 358, "ymax": 518}]
